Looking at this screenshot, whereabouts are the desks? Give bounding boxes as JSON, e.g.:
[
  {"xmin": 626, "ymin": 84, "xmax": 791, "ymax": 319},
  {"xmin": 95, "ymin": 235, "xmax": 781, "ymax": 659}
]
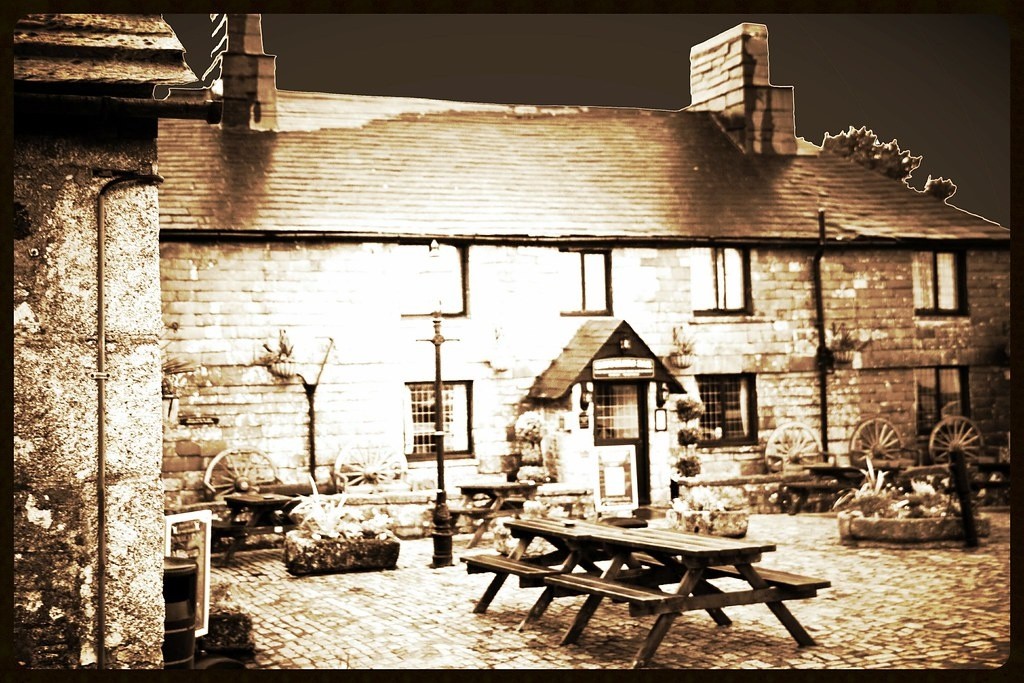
[
  {"xmin": 559, "ymin": 529, "xmax": 818, "ymax": 667},
  {"xmin": 217, "ymin": 494, "xmax": 302, "ymax": 566},
  {"xmin": 454, "ymin": 482, "xmax": 537, "ymax": 548},
  {"xmin": 472, "ymin": 517, "xmax": 629, "ymax": 633}
]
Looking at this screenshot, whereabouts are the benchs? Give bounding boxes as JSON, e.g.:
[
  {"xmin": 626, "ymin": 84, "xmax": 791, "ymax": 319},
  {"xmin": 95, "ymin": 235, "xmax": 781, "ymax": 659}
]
[
  {"xmin": 708, "ymin": 565, "xmax": 832, "ymax": 591},
  {"xmin": 458, "ymin": 549, "xmax": 678, "ymax": 589},
  {"xmin": 427, "ymin": 497, "xmax": 528, "ymax": 520},
  {"xmin": 211, "ymin": 519, "xmax": 245, "ymax": 537},
  {"xmin": 543, "ymin": 572, "xmax": 683, "ymax": 617}
]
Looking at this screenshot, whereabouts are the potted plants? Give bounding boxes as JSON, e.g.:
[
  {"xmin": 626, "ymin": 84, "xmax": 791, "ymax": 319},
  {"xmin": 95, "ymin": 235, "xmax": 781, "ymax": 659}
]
[{"xmin": 670, "ymin": 324, "xmax": 694, "ymax": 369}]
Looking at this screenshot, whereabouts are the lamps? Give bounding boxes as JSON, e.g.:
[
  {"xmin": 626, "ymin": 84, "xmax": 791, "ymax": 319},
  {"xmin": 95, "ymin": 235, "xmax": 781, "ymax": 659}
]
[{"xmin": 659, "ymin": 387, "xmax": 669, "ymax": 403}]
